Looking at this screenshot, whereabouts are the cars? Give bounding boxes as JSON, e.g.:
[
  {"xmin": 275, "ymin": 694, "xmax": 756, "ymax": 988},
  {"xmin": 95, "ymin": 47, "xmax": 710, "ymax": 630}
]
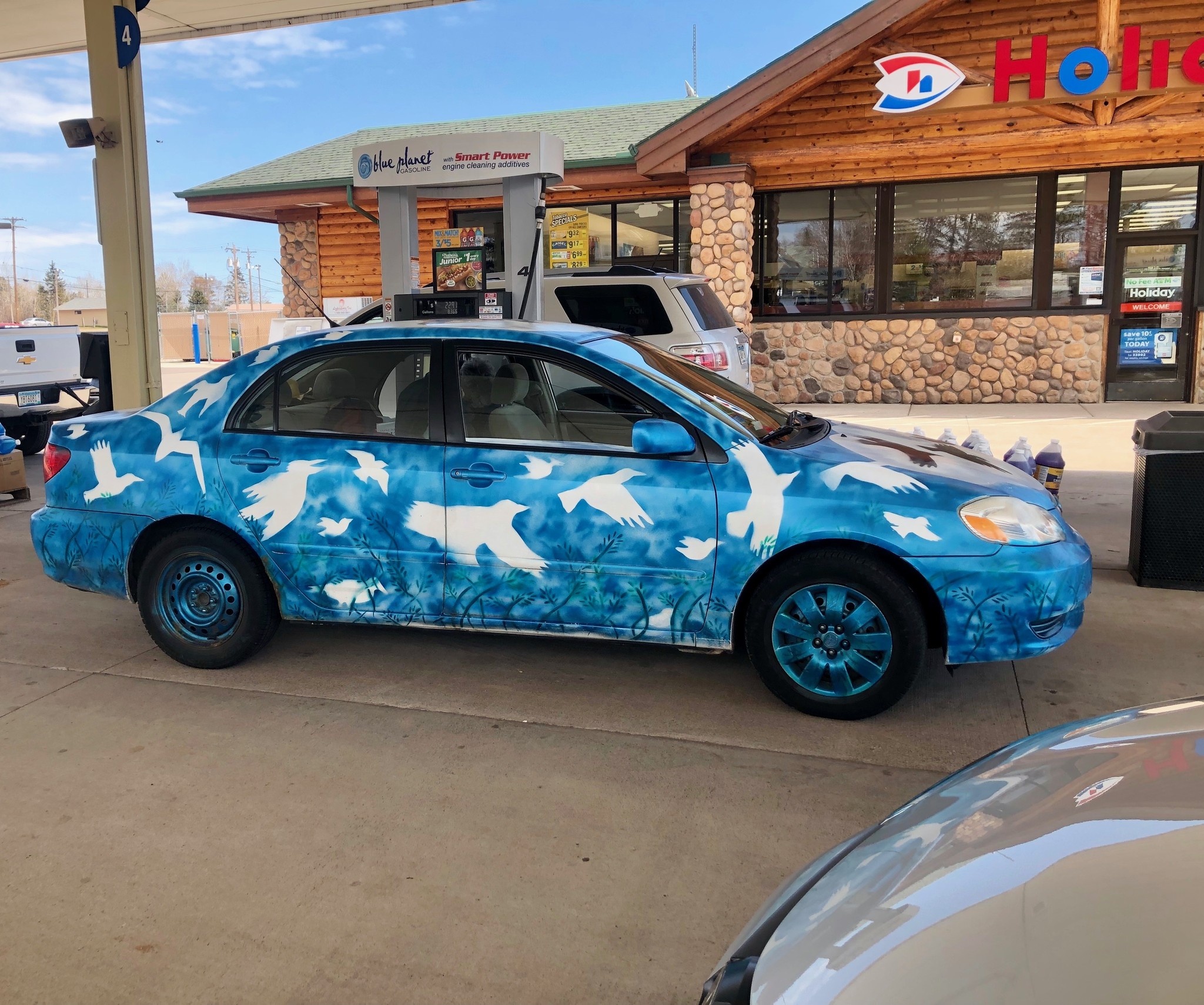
[
  {"xmin": 19, "ymin": 319, "xmax": 52, "ymax": 327},
  {"xmin": 25, "ymin": 315, "xmax": 1096, "ymax": 721},
  {"xmin": 700, "ymin": 689, "xmax": 1204, "ymax": 1003},
  {"xmin": 0, "ymin": 322, "xmax": 25, "ymax": 328},
  {"xmin": 778, "ymin": 289, "xmax": 862, "ymax": 314}
]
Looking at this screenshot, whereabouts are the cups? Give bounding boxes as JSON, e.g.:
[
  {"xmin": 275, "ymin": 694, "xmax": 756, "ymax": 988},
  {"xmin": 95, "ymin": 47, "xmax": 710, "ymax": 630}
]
[{"xmin": 442, "ymin": 265, "xmax": 451, "ymax": 271}]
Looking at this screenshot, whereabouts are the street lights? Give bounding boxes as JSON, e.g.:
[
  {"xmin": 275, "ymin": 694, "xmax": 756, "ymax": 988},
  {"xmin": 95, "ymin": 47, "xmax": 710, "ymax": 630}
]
[{"xmin": 0, "ymin": 215, "xmax": 27, "ymax": 321}]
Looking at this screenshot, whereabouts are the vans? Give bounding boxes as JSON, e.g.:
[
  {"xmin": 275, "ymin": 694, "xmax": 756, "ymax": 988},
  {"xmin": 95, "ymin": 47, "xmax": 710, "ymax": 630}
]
[{"xmin": 327, "ymin": 259, "xmax": 753, "ymax": 420}]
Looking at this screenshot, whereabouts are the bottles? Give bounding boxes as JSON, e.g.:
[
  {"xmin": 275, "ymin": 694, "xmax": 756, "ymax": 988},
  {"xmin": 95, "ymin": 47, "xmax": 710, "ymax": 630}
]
[
  {"xmin": 588, "ymin": 236, "xmax": 600, "ymax": 260},
  {"xmin": 0, "ymin": 422, "xmax": 21, "ymax": 455},
  {"xmin": 909, "ymin": 424, "xmax": 1065, "ymax": 498},
  {"xmin": 460, "ymin": 227, "xmax": 484, "ymax": 247}
]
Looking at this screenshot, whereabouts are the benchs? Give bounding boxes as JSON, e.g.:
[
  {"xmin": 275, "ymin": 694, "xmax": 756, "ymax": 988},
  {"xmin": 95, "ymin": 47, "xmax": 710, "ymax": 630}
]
[{"xmin": 244, "ymin": 368, "xmax": 384, "ymax": 434}]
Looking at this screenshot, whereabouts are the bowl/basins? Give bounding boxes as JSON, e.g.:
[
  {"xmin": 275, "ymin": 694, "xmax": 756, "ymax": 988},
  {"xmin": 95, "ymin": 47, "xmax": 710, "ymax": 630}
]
[
  {"xmin": 471, "ymin": 260, "xmax": 482, "ymax": 271},
  {"xmin": 476, "ymin": 271, "xmax": 482, "ymax": 283},
  {"xmin": 465, "ymin": 275, "xmax": 477, "ymax": 288}
]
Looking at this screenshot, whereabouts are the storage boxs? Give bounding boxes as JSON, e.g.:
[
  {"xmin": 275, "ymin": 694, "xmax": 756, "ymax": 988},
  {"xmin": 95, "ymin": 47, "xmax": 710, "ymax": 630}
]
[{"xmin": 0, "ymin": 449, "xmax": 27, "ymax": 492}]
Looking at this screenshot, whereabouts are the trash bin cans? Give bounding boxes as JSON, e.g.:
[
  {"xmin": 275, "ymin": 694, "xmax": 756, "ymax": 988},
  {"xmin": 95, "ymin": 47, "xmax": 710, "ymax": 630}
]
[{"xmin": 1131, "ymin": 411, "xmax": 1204, "ymax": 596}]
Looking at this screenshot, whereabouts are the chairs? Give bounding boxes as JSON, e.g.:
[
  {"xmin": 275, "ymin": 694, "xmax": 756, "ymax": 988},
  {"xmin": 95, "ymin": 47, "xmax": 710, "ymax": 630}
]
[
  {"xmin": 487, "ymin": 363, "xmax": 556, "ymax": 443},
  {"xmin": 394, "ymin": 370, "xmax": 464, "ymax": 441},
  {"xmin": 461, "ymin": 357, "xmax": 497, "ymax": 440}
]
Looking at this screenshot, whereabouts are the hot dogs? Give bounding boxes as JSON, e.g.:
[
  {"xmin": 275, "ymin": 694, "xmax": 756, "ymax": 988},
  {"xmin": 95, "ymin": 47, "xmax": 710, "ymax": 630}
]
[{"xmin": 438, "ymin": 264, "xmax": 471, "ymax": 289}]
